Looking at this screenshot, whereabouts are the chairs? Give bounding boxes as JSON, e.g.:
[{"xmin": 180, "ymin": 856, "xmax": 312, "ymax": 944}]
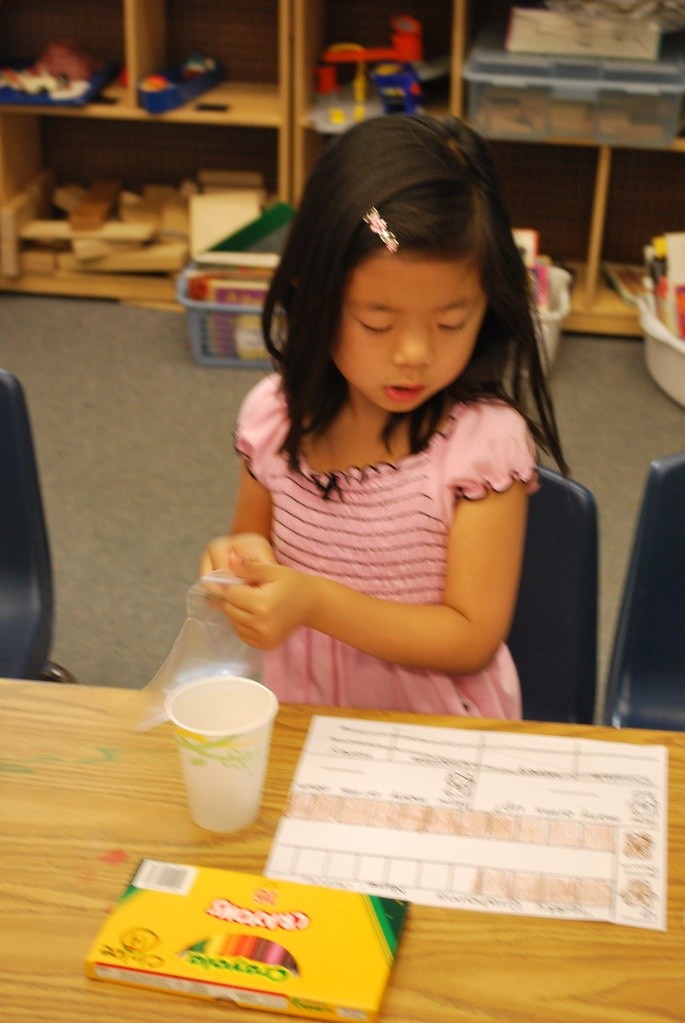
[
  {"xmin": 599, "ymin": 457, "xmax": 684, "ymax": 730},
  {"xmin": 504, "ymin": 465, "xmax": 601, "ymax": 725},
  {"xmin": 0, "ymin": 369, "xmax": 74, "ymax": 685}
]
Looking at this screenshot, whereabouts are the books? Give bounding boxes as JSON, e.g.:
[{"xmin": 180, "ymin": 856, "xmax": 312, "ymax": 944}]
[
  {"xmin": 603, "ymin": 263, "xmax": 647, "ymax": 304},
  {"xmin": 184, "ymin": 268, "xmax": 282, "ymax": 357},
  {"xmin": 512, "ymin": 228, "xmax": 548, "ymax": 313}
]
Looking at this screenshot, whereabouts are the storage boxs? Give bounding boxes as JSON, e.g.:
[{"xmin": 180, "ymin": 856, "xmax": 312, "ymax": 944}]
[
  {"xmin": 86, "ymin": 859, "xmax": 411, "ymax": 1023},
  {"xmin": 504, "ymin": 7, "xmax": 662, "ymax": 62},
  {"xmin": 463, "ymin": 24, "xmax": 684, "ymax": 149},
  {"xmin": 526, "ymin": 267, "xmax": 573, "ymax": 377},
  {"xmin": 632, "ymin": 294, "xmax": 685, "ymax": 408},
  {"xmin": 177, "ymin": 269, "xmax": 283, "ymax": 370}
]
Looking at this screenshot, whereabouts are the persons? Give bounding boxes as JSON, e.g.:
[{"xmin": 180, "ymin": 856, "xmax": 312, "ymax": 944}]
[{"xmin": 198, "ymin": 116, "xmax": 572, "ymax": 726}]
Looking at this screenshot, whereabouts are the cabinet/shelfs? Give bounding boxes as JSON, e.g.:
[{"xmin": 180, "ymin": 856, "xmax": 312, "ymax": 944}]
[
  {"xmin": 291, "ymin": 0, "xmax": 684, "ymax": 338},
  {"xmin": 1, "ymin": 1, "xmax": 291, "ymax": 309}
]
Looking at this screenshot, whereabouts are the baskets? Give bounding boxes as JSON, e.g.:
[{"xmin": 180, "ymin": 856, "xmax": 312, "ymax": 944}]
[{"xmin": 177, "ymin": 257, "xmax": 285, "ymax": 370}]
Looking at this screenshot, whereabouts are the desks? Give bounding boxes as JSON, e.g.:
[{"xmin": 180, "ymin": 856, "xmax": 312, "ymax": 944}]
[{"xmin": 1, "ymin": 677, "xmax": 685, "ymax": 1023}]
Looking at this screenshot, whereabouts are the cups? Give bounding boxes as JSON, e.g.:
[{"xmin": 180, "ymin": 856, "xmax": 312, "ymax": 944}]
[{"xmin": 169, "ymin": 674, "xmax": 279, "ymax": 827}]
